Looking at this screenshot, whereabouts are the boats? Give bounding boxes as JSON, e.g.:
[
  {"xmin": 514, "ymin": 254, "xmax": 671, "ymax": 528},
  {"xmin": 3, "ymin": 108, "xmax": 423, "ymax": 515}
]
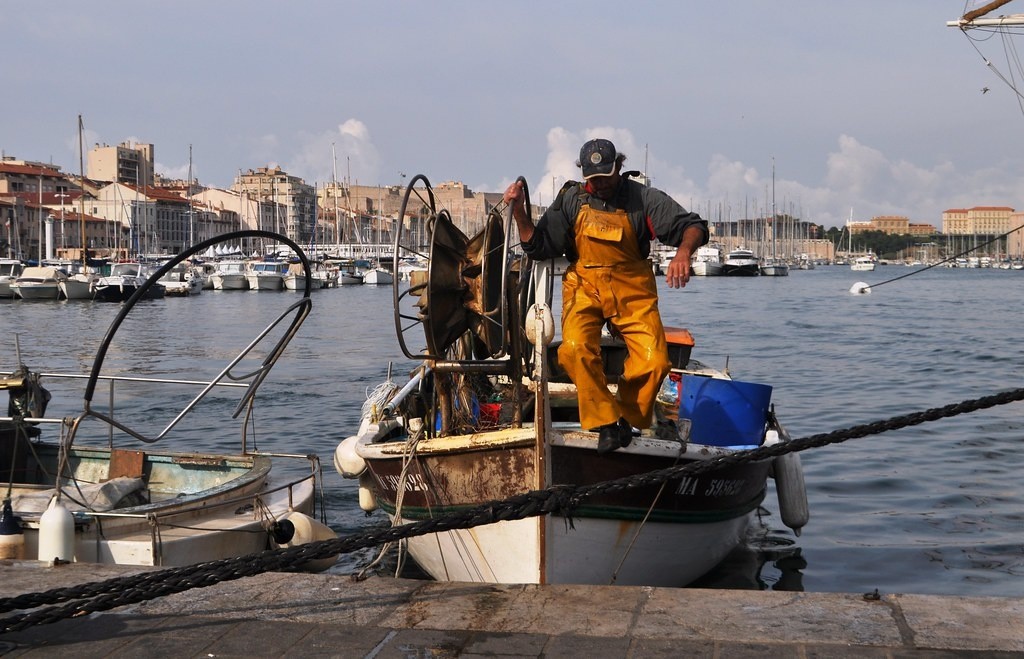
[
  {"xmin": 1, "ymin": 230, "xmax": 341, "ymax": 576},
  {"xmin": 332, "ymin": 174, "xmax": 811, "ymax": 586},
  {"xmin": 850, "ymin": 257, "xmax": 875, "ymax": 271}
]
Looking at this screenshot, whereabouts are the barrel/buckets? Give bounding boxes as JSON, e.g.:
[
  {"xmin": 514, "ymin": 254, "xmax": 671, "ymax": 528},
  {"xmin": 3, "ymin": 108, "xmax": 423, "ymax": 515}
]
[{"xmin": 678, "ymin": 373, "xmax": 773, "ymax": 447}]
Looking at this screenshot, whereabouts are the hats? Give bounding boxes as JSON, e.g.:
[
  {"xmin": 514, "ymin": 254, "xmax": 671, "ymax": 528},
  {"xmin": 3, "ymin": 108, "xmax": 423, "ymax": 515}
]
[{"xmin": 579, "ymin": 139, "xmax": 618, "ymax": 180}]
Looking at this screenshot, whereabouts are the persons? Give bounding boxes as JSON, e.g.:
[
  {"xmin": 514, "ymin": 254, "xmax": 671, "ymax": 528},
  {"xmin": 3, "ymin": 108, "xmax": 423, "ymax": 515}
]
[{"xmin": 504, "ymin": 139, "xmax": 710, "ymax": 454}]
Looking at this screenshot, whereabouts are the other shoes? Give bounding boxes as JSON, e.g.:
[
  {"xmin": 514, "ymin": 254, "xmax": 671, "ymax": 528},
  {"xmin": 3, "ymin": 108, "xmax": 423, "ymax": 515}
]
[
  {"xmin": 598, "ymin": 421, "xmax": 620, "ymax": 453},
  {"xmin": 617, "ymin": 417, "xmax": 632, "ymax": 448}
]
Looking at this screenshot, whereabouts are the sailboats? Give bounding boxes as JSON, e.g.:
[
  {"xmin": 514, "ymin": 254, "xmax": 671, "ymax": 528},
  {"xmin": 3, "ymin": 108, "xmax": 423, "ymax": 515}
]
[
  {"xmin": 645, "ymin": 143, "xmax": 851, "ymax": 277},
  {"xmin": 894, "ymin": 229, "xmax": 1024, "ymax": 270},
  {"xmin": 1, "ymin": 115, "xmax": 431, "ymax": 301}
]
